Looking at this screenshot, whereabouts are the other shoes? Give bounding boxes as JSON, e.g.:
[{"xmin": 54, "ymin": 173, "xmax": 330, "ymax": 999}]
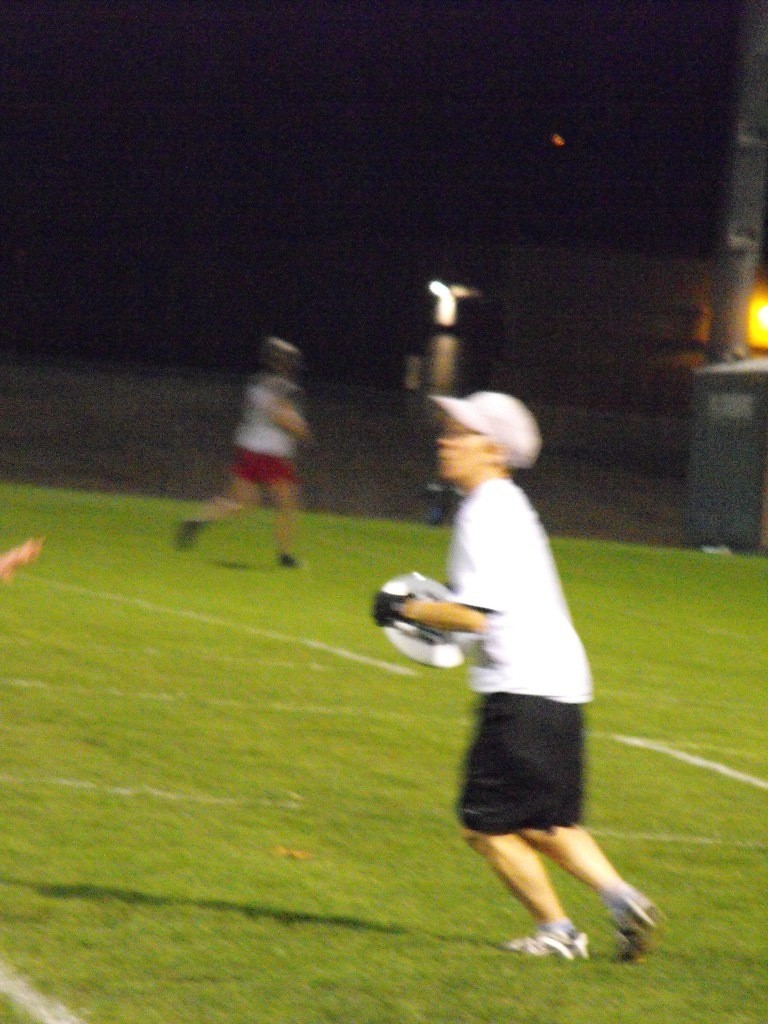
[
  {"xmin": 279, "ymin": 555, "xmax": 299, "ymax": 568},
  {"xmin": 180, "ymin": 518, "xmax": 200, "ymax": 542}
]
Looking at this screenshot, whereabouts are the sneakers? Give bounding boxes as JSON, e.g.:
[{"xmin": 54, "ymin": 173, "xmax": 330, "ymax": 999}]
[
  {"xmin": 618, "ymin": 894, "xmax": 663, "ymax": 961},
  {"xmin": 507, "ymin": 932, "xmax": 591, "ymax": 959}
]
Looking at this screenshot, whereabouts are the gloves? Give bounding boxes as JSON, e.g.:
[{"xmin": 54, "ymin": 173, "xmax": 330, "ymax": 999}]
[{"xmin": 373, "ymin": 591, "xmax": 415, "ymax": 626}]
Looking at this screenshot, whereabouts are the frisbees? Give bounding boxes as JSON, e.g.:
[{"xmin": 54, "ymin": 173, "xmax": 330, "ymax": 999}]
[{"xmin": 376, "ymin": 572, "xmax": 466, "ymax": 670}]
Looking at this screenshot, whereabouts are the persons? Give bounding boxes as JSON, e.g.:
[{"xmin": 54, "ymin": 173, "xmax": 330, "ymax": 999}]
[
  {"xmin": 1, "ymin": 534, "xmax": 44, "ymax": 580},
  {"xmin": 371, "ymin": 390, "xmax": 668, "ymax": 967},
  {"xmin": 173, "ymin": 335, "xmax": 309, "ymax": 571}
]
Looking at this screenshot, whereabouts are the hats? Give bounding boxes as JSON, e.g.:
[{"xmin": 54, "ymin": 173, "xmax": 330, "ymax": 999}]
[{"xmin": 427, "ymin": 390, "xmax": 541, "ymax": 468}]
